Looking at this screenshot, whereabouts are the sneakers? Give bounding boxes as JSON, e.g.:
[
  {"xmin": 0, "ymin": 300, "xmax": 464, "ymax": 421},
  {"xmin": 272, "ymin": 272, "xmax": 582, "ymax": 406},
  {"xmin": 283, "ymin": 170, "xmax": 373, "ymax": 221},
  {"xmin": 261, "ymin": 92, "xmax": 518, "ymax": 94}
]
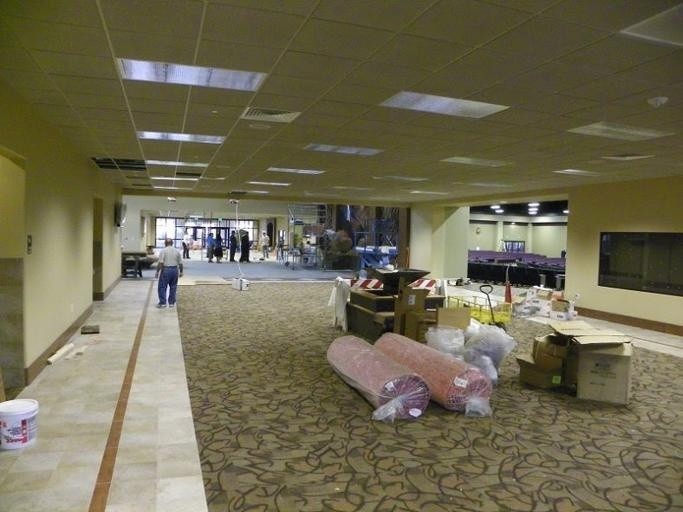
[
  {"xmin": 169, "ymin": 303, "xmax": 174, "ymax": 307},
  {"xmin": 157, "ymin": 304, "xmax": 166, "ymax": 308}
]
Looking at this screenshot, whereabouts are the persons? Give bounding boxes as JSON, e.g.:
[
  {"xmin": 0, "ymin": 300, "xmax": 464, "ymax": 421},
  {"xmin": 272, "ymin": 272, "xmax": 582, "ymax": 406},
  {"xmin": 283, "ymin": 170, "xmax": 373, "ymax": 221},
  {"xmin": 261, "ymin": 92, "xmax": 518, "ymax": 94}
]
[
  {"xmin": 206, "ymin": 229, "xmax": 250, "ymax": 263},
  {"xmin": 155, "ymin": 238, "xmax": 184, "ymax": 308},
  {"xmin": 182, "ymin": 238, "xmax": 191, "ymax": 259},
  {"xmin": 147, "ymin": 246, "xmax": 154, "ymax": 255},
  {"xmin": 262, "ymin": 232, "xmax": 270, "ymax": 259}
]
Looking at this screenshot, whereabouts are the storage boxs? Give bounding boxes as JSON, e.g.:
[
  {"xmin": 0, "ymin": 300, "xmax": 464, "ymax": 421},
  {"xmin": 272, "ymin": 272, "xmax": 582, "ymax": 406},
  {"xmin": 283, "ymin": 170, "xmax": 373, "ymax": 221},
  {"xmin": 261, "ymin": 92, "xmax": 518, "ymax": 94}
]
[
  {"xmin": 516, "ymin": 319, "xmax": 633, "ymax": 406},
  {"xmin": 395, "ymin": 287, "xmax": 470, "ymax": 345}
]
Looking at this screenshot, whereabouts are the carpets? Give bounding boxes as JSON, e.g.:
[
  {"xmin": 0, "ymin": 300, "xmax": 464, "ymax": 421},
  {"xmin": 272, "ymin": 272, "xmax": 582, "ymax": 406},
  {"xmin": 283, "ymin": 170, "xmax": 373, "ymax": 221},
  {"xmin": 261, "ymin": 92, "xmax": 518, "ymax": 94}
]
[{"xmin": 175, "ymin": 283, "xmax": 682, "ymax": 510}]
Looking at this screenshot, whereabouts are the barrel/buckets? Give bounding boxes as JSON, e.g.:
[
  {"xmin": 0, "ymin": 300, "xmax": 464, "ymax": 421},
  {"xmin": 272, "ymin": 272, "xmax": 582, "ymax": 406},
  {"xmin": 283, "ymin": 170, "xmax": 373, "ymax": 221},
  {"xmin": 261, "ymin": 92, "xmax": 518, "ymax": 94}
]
[{"xmin": 0, "ymin": 398, "xmax": 40, "ymax": 450}]
